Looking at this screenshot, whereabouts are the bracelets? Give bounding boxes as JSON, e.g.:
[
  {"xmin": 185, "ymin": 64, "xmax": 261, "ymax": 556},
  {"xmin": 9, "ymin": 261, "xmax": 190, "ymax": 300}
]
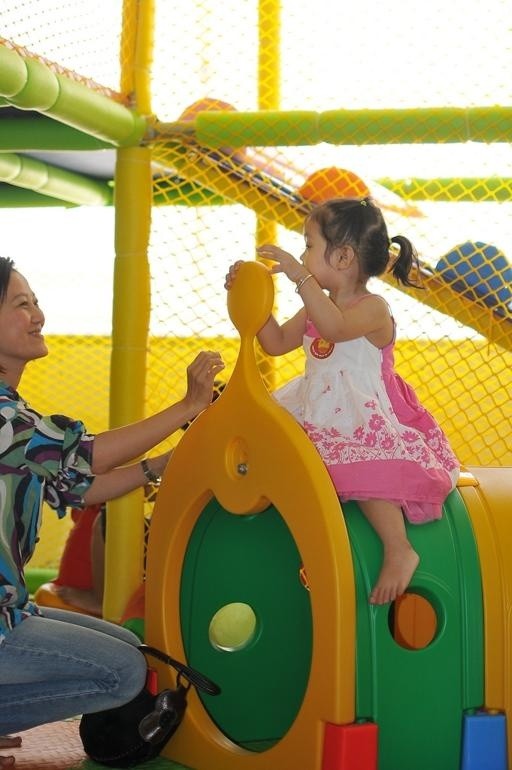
[
  {"xmin": 141, "ymin": 460, "xmax": 156, "ymax": 482},
  {"xmin": 295, "ymin": 274, "xmax": 313, "ymax": 293}
]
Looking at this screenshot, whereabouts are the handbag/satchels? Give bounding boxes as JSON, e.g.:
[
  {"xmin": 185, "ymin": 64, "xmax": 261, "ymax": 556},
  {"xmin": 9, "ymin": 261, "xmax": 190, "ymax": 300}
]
[{"xmin": 78, "ymin": 645, "xmax": 222, "ymax": 769}]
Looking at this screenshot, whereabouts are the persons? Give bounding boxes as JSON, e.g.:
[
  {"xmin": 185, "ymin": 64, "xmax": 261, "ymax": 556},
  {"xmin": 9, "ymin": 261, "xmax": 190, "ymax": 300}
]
[
  {"xmin": 224, "ymin": 197, "xmax": 460, "ymax": 606},
  {"xmin": 0, "ymin": 257, "xmax": 225, "ymax": 770}
]
